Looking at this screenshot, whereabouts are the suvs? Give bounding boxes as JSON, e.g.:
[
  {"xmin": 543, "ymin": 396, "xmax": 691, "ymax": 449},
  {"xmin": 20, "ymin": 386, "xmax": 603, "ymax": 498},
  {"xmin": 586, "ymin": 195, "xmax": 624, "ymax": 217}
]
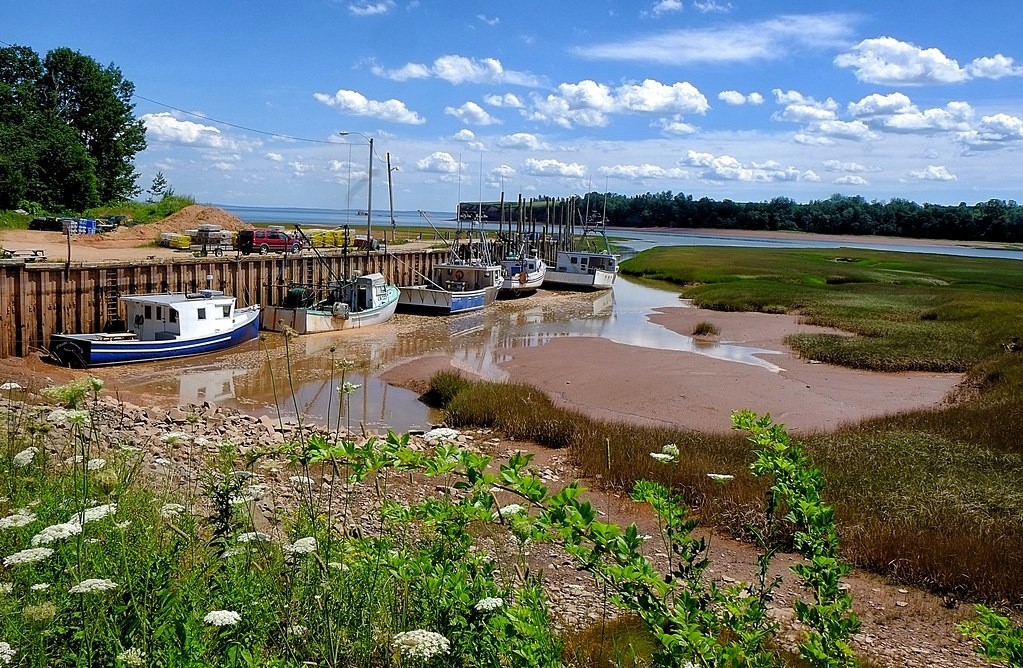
[{"xmin": 236, "ymin": 228, "xmax": 303, "ymax": 256}]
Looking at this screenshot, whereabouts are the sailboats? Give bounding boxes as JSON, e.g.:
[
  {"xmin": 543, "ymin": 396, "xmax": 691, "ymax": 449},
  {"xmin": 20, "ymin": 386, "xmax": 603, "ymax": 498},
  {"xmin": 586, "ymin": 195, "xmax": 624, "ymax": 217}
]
[
  {"xmin": 481, "ymin": 173, "xmax": 547, "ymax": 297},
  {"xmin": 546, "ymin": 175, "xmax": 621, "ymax": 293},
  {"xmin": 397, "ymin": 153, "xmax": 503, "ymax": 316}
]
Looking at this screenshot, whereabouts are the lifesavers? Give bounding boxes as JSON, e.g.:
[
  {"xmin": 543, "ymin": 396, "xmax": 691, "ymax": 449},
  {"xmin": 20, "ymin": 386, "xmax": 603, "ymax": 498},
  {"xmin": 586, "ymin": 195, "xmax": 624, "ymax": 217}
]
[
  {"xmin": 135, "ymin": 315, "xmax": 139, "ymax": 324},
  {"xmin": 138, "ymin": 314, "xmax": 144, "ymax": 325},
  {"xmin": 455, "ymin": 270, "xmax": 464, "ymax": 280}
]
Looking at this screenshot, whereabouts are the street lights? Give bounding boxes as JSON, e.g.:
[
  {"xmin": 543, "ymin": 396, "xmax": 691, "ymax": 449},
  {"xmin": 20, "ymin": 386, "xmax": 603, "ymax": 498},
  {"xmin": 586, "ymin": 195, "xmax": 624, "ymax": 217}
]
[{"xmin": 339, "ymin": 131, "xmax": 373, "ymax": 275}]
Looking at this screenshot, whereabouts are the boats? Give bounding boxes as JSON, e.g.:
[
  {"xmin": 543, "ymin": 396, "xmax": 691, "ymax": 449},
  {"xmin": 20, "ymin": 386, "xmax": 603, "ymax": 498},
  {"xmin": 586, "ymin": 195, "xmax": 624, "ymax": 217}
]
[
  {"xmin": 262, "ymin": 224, "xmax": 401, "ymax": 337},
  {"xmin": 35, "ymin": 273, "xmax": 261, "ymax": 370}
]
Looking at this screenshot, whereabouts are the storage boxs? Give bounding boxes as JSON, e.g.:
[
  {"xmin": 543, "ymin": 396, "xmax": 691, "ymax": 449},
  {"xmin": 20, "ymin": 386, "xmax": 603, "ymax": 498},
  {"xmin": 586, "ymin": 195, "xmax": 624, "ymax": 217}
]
[
  {"xmin": 62, "ymin": 217, "xmax": 96, "ymax": 234},
  {"xmin": 161, "ymin": 230, "xmax": 239, "ymax": 250},
  {"xmin": 301, "ymin": 229, "xmax": 356, "ymax": 248}
]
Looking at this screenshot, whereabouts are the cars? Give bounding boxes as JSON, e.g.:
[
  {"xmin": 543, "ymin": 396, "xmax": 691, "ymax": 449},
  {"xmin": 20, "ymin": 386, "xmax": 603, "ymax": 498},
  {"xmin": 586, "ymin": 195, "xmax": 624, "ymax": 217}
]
[
  {"xmin": 28, "ymin": 214, "xmax": 127, "ymax": 236},
  {"xmin": 354, "ymin": 234, "xmax": 378, "ymax": 249}
]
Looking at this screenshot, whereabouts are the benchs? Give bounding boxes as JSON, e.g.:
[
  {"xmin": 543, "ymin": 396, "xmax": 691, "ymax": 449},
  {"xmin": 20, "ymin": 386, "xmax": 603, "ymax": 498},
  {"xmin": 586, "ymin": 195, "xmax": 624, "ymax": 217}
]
[{"xmin": 1, "ymin": 253, "xmax": 47, "ymax": 259}]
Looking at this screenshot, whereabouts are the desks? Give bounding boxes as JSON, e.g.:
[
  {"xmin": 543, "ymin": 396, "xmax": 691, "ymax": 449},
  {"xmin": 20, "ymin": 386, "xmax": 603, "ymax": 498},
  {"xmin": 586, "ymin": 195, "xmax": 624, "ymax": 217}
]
[
  {"xmin": 4, "ymin": 249, "xmax": 46, "ymax": 262},
  {"xmin": 97, "ymin": 333, "xmax": 137, "ymax": 340}
]
